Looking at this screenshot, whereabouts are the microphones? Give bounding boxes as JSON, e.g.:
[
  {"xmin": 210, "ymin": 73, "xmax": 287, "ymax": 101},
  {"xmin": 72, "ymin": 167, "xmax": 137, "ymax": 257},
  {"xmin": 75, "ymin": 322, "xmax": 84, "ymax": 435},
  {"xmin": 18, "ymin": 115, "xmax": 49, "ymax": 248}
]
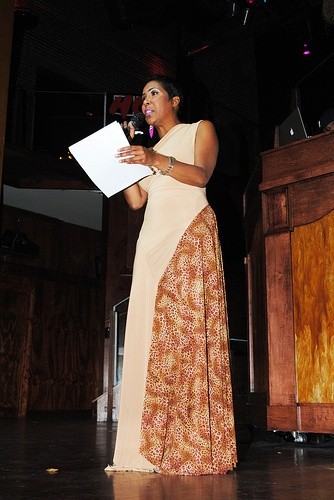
[{"xmin": 125, "ymin": 113, "xmax": 145, "ymax": 137}]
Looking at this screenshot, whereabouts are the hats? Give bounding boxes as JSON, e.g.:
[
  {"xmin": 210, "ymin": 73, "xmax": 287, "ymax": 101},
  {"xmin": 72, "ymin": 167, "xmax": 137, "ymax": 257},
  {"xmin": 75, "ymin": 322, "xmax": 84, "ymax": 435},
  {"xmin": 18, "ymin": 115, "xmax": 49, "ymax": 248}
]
[{"xmin": 310, "ymin": 111, "xmax": 334, "ymax": 130}]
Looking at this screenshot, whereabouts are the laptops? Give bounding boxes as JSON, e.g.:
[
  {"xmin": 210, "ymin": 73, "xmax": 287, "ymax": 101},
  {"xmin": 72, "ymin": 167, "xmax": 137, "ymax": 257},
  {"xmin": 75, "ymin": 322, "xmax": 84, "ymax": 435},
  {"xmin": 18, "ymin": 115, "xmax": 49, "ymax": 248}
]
[{"xmin": 279, "ymin": 106, "xmax": 308, "ymax": 146}]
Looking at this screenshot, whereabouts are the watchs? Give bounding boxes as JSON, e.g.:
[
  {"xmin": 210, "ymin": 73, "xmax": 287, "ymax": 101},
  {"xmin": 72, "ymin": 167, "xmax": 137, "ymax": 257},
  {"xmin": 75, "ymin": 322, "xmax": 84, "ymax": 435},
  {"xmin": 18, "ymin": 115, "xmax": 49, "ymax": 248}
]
[{"xmin": 158, "ymin": 156, "xmax": 176, "ymax": 176}]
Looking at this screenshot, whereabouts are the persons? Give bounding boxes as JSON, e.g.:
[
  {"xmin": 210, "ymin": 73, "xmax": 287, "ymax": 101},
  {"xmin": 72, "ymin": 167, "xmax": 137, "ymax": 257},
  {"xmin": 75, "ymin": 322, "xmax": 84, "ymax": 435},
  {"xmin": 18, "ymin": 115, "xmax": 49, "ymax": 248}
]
[{"xmin": 104, "ymin": 74, "xmax": 238, "ymax": 477}]
